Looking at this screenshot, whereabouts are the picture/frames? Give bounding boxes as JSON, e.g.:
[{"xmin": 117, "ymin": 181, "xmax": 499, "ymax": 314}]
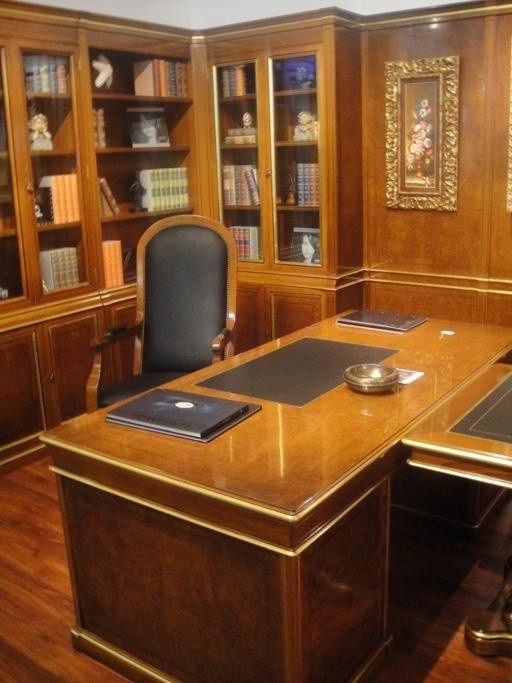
[{"xmin": 382, "ymin": 52, "xmax": 463, "ymax": 213}]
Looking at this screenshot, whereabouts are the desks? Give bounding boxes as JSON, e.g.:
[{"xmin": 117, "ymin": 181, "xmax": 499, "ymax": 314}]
[{"xmin": 37, "ymin": 311, "xmax": 512, "ymax": 683}]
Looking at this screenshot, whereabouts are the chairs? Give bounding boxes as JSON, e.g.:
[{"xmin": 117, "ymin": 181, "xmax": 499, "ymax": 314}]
[{"xmin": 85, "ymin": 213, "xmax": 240, "ymax": 411}]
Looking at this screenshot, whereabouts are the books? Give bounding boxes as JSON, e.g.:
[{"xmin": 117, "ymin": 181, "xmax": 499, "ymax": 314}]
[
  {"xmin": 91, "ymin": 59, "xmax": 186, "ymax": 288},
  {"xmin": 218, "ymin": 65, "xmax": 321, "ymax": 264},
  {"xmin": 22, "ymin": 51, "xmax": 82, "ymax": 292}
]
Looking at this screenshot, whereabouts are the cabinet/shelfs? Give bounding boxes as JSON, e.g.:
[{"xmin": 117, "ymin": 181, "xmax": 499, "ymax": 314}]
[
  {"xmin": 2, "ymin": 0, "xmax": 201, "ymax": 457},
  {"xmin": 196, "ymin": 5, "xmax": 361, "ymax": 358}
]
